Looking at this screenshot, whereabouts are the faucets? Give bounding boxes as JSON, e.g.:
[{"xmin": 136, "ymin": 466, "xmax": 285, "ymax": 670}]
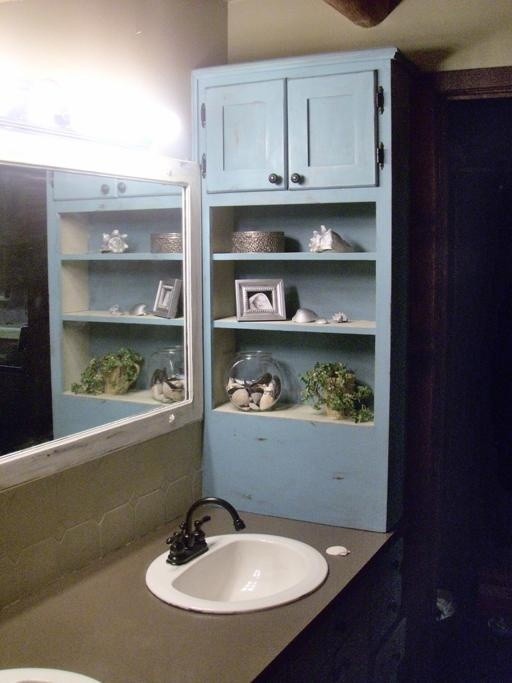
[{"xmin": 184, "ymin": 495, "xmax": 245, "ymax": 536}]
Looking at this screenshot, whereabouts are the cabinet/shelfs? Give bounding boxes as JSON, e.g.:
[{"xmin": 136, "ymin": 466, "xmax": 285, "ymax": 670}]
[
  {"xmin": 204, "ymin": 193, "xmax": 390, "ymax": 529},
  {"xmin": 56, "ymin": 205, "xmax": 185, "ymax": 409},
  {"xmin": 363, "ymin": 536, "xmax": 408, "ymax": 682},
  {"xmin": 197, "ymin": 68, "xmax": 380, "ymax": 193},
  {"xmin": 52, "ymin": 169, "xmax": 183, "ymax": 201}
]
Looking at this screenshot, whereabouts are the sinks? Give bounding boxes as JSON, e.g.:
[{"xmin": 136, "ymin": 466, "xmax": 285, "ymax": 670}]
[
  {"xmin": 0, "ymin": 667, "xmax": 102, "ymax": 683},
  {"xmin": 144, "ymin": 533, "xmax": 328, "ymax": 614}
]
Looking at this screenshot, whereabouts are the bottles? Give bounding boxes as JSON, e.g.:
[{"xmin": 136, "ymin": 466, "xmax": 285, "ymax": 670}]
[
  {"xmin": 148, "ymin": 346, "xmax": 185, "ymax": 404},
  {"xmin": 223, "ymin": 351, "xmax": 284, "ymax": 411}
]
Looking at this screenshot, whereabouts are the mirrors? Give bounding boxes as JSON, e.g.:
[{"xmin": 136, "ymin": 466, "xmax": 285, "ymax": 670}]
[{"xmin": 0, "ymin": 119, "xmax": 206, "ymax": 490}]
